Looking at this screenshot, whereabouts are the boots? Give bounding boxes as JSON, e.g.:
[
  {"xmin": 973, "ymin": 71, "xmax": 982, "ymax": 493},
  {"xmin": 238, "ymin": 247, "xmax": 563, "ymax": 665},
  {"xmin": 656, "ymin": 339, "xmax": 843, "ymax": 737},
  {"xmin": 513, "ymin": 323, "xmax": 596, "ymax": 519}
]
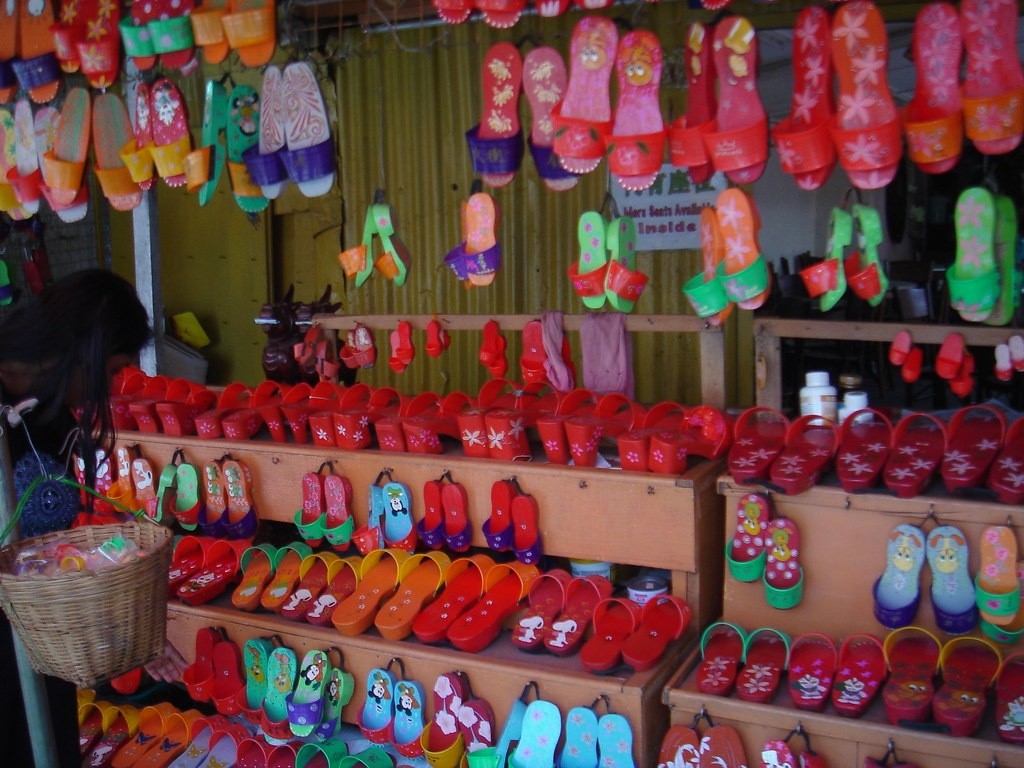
[{"xmin": 66, "ymin": 319, "xmax": 1024, "ymax": 768}]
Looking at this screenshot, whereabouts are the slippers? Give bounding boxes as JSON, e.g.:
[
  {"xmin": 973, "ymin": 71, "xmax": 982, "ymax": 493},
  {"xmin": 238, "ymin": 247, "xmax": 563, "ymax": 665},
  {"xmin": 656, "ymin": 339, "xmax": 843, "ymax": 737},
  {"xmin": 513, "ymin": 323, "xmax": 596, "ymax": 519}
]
[
  {"xmin": 1, "ymin": 0, "xmax": 336, "ymax": 223},
  {"xmin": 434, "ymin": 2, "xmax": 1023, "ymax": 325},
  {"xmin": 336, "ymin": 204, "xmax": 413, "ymax": 288},
  {"xmin": 0, "ymin": 223, "xmax": 52, "ymax": 305}
]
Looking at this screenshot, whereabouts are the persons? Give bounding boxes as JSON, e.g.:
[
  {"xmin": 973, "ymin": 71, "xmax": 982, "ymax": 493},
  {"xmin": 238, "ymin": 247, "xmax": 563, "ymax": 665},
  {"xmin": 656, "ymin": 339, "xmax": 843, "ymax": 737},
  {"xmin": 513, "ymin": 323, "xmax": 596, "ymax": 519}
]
[{"xmin": 0, "ymin": 267, "xmax": 153, "ymax": 768}]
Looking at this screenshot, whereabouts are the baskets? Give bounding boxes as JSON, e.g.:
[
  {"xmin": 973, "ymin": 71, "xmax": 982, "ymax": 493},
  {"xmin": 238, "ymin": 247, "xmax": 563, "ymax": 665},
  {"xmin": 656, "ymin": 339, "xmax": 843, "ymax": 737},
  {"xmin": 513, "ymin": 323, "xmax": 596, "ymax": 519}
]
[{"xmin": 2, "ymin": 525, "xmax": 174, "ymax": 687}]
